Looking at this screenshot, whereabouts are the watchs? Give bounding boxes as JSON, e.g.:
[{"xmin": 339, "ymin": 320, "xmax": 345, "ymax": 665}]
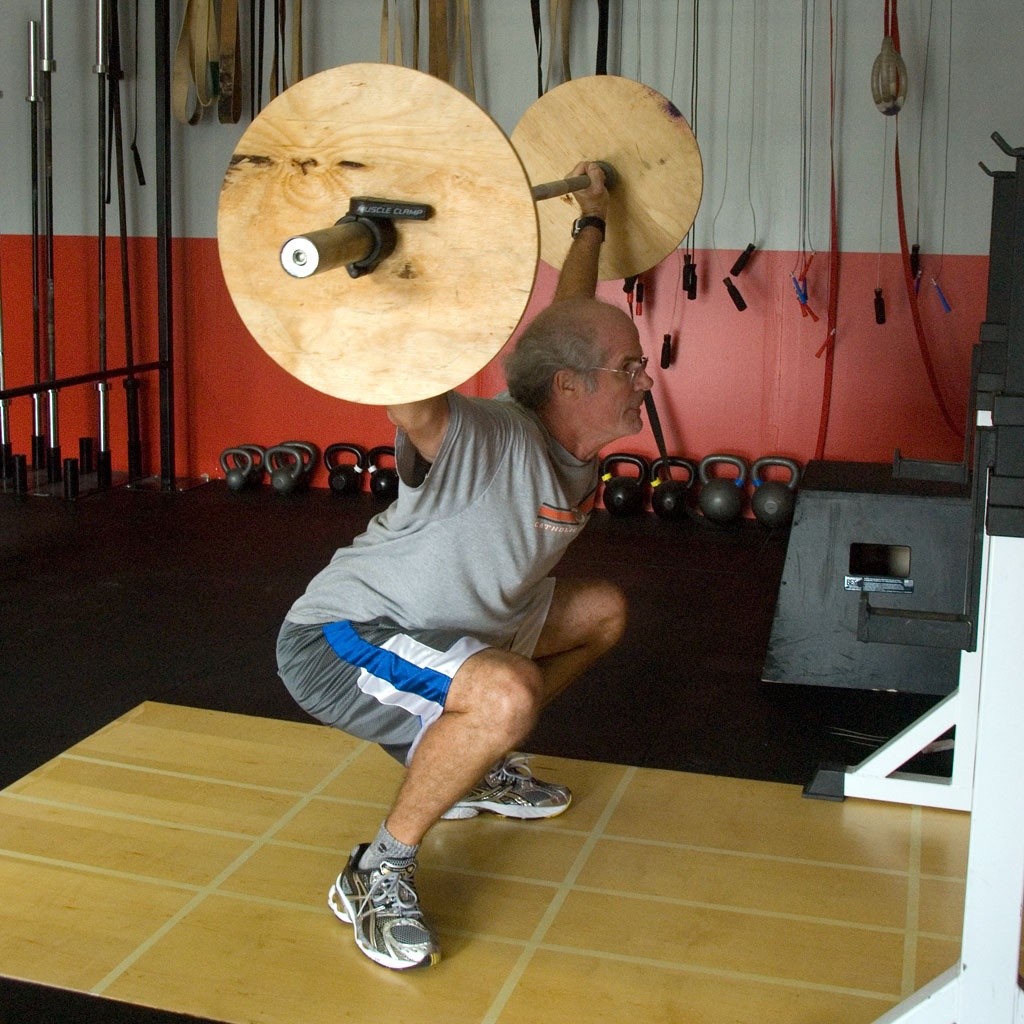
[{"xmin": 570, "ymin": 216, "xmax": 606, "ymax": 243}]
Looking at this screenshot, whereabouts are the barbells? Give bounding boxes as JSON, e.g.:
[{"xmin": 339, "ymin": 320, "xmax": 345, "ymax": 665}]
[{"xmin": 217, "ymin": 60, "xmax": 704, "ymax": 406}]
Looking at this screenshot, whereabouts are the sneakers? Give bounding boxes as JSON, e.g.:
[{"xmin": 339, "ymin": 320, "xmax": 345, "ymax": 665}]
[
  {"xmin": 437, "ymin": 757, "xmax": 572, "ymax": 818},
  {"xmin": 327, "ymin": 842, "xmax": 443, "ymax": 970}
]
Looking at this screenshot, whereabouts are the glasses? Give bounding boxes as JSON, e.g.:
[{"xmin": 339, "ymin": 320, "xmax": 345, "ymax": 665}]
[{"xmin": 568, "ymin": 354, "xmax": 649, "ymax": 383}]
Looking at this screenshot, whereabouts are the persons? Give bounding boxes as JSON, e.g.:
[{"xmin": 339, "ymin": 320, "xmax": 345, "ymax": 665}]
[{"xmin": 277, "ymin": 160, "xmax": 656, "ymax": 964}]
